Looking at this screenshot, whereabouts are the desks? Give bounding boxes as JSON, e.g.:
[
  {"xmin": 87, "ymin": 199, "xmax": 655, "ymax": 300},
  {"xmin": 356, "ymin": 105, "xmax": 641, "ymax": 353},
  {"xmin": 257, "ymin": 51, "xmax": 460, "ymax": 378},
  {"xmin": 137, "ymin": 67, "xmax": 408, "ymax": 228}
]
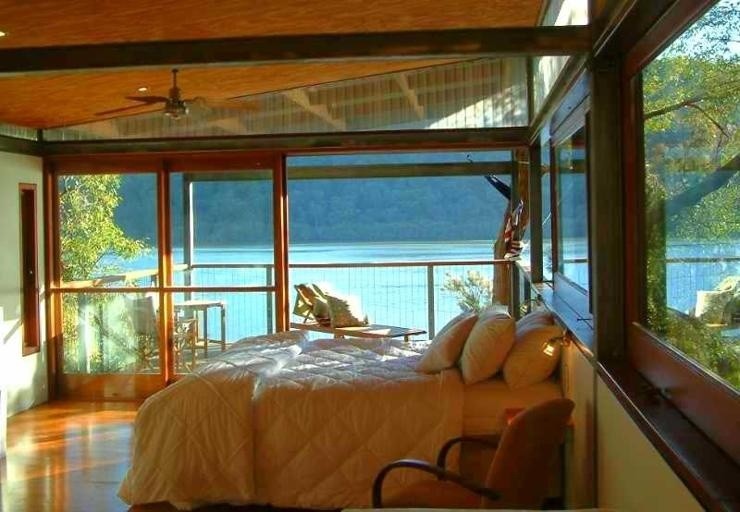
[{"xmin": 172, "ymin": 299, "xmax": 232, "ymax": 353}]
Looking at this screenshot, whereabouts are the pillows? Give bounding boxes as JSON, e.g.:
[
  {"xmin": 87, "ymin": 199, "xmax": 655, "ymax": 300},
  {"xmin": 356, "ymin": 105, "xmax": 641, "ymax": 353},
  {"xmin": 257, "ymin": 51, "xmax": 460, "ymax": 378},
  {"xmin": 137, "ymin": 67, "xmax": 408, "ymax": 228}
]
[{"xmin": 414, "ymin": 303, "xmax": 561, "ymax": 389}]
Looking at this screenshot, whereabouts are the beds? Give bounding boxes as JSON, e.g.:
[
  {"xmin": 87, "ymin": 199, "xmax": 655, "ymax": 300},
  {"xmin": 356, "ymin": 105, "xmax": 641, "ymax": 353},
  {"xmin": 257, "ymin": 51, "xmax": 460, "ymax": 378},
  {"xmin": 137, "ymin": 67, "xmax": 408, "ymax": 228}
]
[{"xmin": 118, "ymin": 322, "xmax": 594, "ymax": 505}]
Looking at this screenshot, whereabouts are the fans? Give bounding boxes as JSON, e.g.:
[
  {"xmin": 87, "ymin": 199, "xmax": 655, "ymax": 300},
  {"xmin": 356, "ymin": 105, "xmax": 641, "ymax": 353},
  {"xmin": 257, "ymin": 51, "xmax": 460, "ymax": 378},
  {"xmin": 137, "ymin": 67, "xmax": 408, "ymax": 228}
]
[{"xmin": 97, "ymin": 68, "xmax": 259, "ymax": 120}]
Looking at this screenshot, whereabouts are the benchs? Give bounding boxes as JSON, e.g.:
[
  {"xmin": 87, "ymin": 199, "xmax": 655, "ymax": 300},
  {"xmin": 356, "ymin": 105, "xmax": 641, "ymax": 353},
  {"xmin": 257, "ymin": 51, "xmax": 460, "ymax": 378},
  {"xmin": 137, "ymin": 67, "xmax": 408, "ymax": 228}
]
[{"xmin": 293, "ymin": 281, "xmax": 426, "ymax": 348}]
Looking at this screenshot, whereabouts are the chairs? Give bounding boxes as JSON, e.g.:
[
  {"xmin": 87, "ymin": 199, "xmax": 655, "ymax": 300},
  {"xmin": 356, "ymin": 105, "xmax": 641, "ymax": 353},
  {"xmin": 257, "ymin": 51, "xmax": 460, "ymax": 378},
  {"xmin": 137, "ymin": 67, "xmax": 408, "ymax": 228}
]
[
  {"xmin": 126, "ymin": 286, "xmax": 198, "ymax": 361},
  {"xmin": 374, "ymin": 399, "xmax": 575, "ymax": 504}
]
